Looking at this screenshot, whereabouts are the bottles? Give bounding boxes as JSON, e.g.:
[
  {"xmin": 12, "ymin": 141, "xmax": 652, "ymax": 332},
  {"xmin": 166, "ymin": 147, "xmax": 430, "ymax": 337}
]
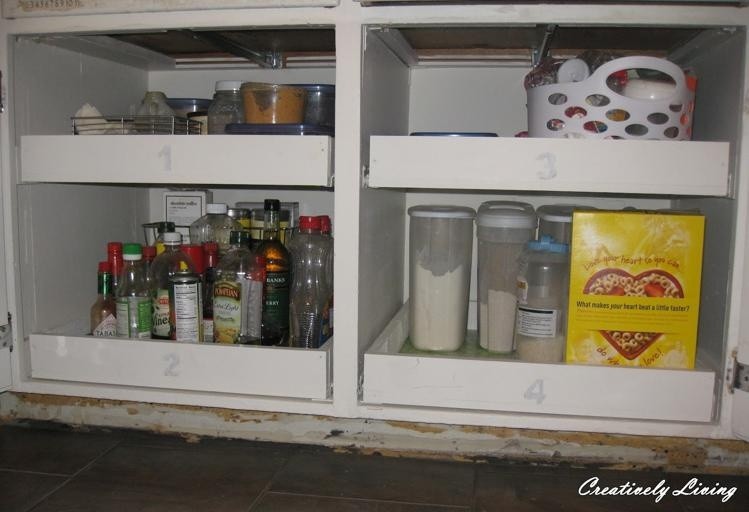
[
  {"xmin": 206, "ymin": 79, "xmax": 245, "ymax": 134},
  {"xmin": 406, "ymin": 204, "xmax": 477, "ymax": 353},
  {"xmin": 538, "ymin": 203, "xmax": 598, "ymax": 242},
  {"xmin": 475, "ymin": 199, "xmax": 536, "ymax": 353},
  {"xmin": 513, "ymin": 240, "xmax": 569, "ymax": 364},
  {"xmin": 90, "ymin": 197, "xmax": 333, "ymax": 347}
]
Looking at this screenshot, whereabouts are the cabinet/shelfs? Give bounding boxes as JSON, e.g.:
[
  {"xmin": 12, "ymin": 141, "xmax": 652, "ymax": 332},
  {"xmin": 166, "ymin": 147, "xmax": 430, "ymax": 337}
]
[{"xmin": 0, "ymin": 0, "xmax": 749, "ymax": 442}]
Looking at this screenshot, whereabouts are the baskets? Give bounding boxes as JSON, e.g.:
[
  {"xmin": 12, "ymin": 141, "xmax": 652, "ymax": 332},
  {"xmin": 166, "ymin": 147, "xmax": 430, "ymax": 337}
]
[
  {"xmin": 526, "ymin": 55, "xmax": 695, "ymax": 140},
  {"xmin": 71, "ymin": 114, "xmax": 204, "ymax": 135}
]
